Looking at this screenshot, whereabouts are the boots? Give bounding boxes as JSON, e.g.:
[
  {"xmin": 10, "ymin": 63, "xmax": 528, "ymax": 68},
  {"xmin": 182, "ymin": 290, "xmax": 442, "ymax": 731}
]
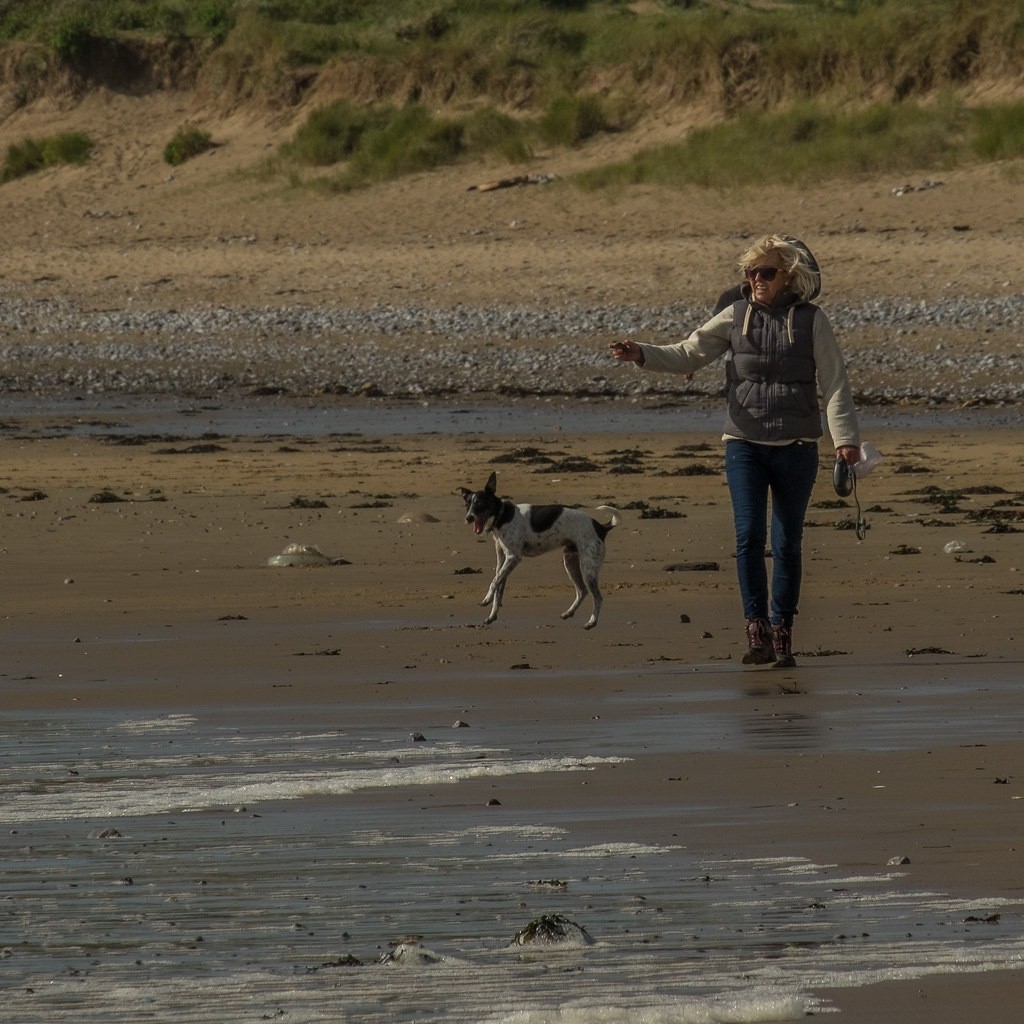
[
  {"xmin": 772, "ymin": 626, "xmax": 796, "ymax": 667},
  {"xmin": 742, "ymin": 620, "xmax": 776, "ymax": 665}
]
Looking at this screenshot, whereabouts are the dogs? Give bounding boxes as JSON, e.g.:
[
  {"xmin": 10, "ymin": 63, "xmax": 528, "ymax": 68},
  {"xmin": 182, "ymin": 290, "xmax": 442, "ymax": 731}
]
[{"xmin": 455, "ymin": 471, "xmax": 622, "ymax": 630}]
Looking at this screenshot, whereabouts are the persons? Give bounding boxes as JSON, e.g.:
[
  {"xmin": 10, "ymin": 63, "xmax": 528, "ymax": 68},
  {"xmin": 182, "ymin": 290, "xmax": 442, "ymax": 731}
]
[{"xmin": 609, "ymin": 232, "xmax": 862, "ymax": 668}]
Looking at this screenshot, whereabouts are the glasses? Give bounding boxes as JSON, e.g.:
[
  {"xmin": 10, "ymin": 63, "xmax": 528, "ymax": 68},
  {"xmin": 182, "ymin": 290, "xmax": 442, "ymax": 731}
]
[{"xmin": 745, "ymin": 267, "xmax": 786, "ymax": 281}]
